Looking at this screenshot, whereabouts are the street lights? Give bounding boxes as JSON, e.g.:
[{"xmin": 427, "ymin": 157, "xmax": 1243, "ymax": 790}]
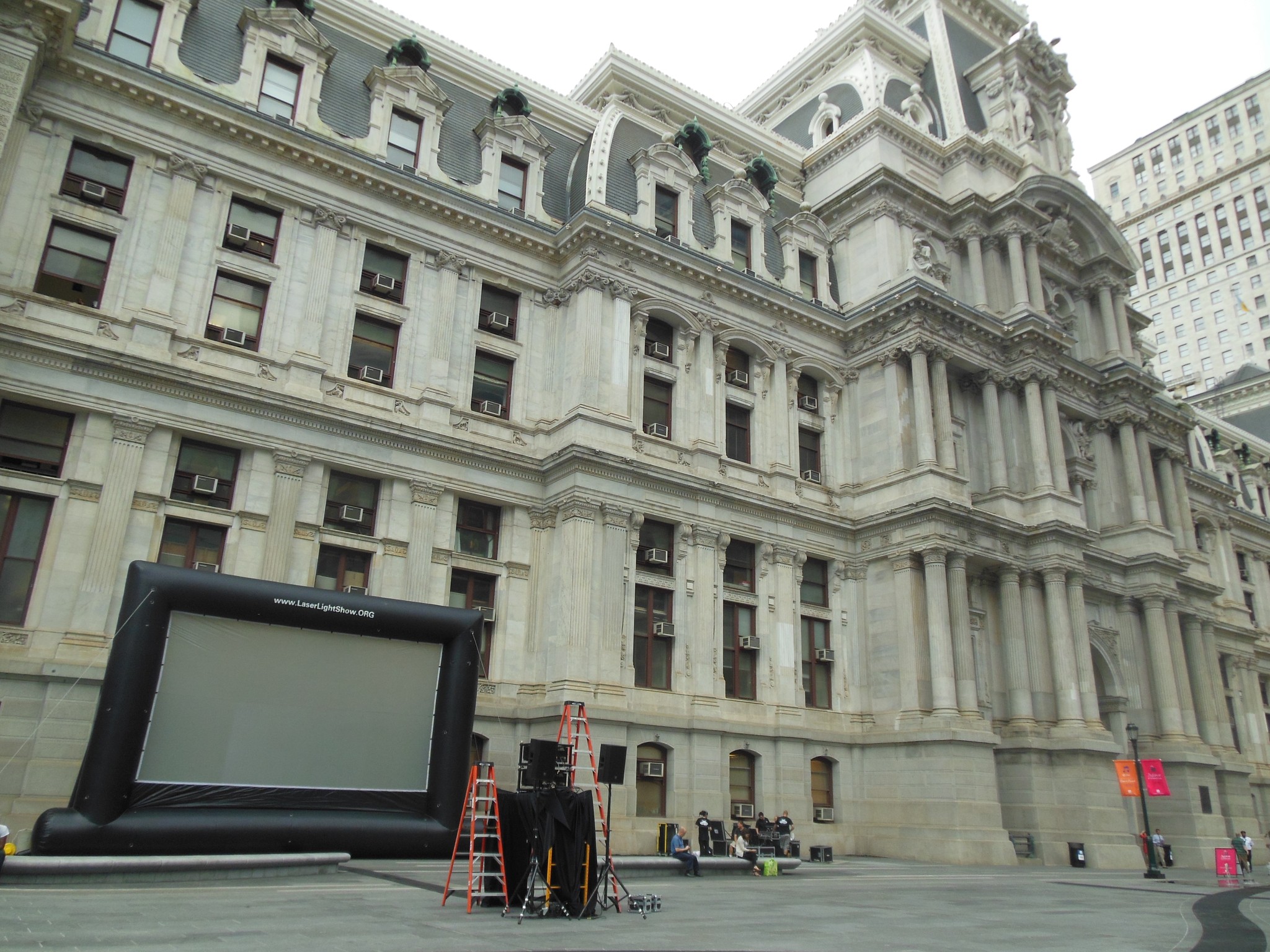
[{"xmin": 1124, "ymin": 724, "xmax": 1166, "ymax": 879}]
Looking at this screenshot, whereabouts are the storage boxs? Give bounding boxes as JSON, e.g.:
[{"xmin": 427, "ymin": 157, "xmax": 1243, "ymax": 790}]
[
  {"xmin": 759, "ymin": 831, "xmax": 780, "ymax": 841},
  {"xmin": 708, "ymin": 819, "xmax": 726, "ymax": 842},
  {"xmin": 745, "ymin": 846, "xmax": 775, "ymax": 858},
  {"xmin": 789, "ymin": 841, "xmax": 800, "ymax": 858},
  {"xmin": 627, "ymin": 894, "xmax": 662, "ymax": 914},
  {"xmin": 810, "ymin": 844, "xmax": 834, "ymax": 864}
]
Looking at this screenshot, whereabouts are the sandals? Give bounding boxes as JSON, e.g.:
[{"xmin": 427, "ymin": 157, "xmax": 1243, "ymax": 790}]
[
  {"xmin": 752, "ymin": 872, "xmax": 761, "ymax": 877},
  {"xmin": 753, "ymin": 867, "xmax": 761, "ymax": 871}
]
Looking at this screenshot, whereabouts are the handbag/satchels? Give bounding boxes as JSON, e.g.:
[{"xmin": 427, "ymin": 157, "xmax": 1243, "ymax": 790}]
[{"xmin": 763, "ymin": 859, "xmax": 777, "ymax": 876}]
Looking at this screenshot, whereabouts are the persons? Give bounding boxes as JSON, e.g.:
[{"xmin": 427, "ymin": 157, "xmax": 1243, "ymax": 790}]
[
  {"xmin": 695, "ymin": 810, "xmax": 795, "ymax": 859},
  {"xmin": 1152, "ymin": 829, "xmax": 1169, "ymax": 869},
  {"xmin": 1230, "ymin": 832, "xmax": 1249, "ymax": 874},
  {"xmin": 671, "ymin": 826, "xmax": 702, "ymax": 877},
  {"xmin": 0, "ymin": 824, "xmax": 10, "ymax": 879},
  {"xmin": 735, "ymin": 829, "xmax": 761, "ymax": 876},
  {"xmin": 1140, "ymin": 830, "xmax": 1150, "ymax": 867},
  {"xmin": 1240, "ymin": 831, "xmax": 1253, "ymax": 872}
]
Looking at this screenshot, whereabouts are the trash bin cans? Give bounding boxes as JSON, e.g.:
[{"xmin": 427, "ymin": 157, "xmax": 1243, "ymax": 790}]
[
  {"xmin": 1163, "ymin": 845, "xmax": 1173, "ymax": 867},
  {"xmin": 1067, "ymin": 841, "xmax": 1085, "ymax": 868}
]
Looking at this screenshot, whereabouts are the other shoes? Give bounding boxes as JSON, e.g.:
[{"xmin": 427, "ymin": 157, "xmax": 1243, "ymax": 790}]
[
  {"xmin": 684, "ymin": 872, "xmax": 694, "ymax": 877},
  {"xmin": 694, "ymin": 871, "xmax": 703, "ymax": 877}
]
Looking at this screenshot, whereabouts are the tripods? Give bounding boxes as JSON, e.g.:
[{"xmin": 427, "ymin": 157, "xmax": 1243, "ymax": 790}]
[
  {"xmin": 577, "ymin": 784, "xmax": 647, "ymax": 921},
  {"xmin": 501, "ymin": 787, "xmax": 573, "ymax": 924}
]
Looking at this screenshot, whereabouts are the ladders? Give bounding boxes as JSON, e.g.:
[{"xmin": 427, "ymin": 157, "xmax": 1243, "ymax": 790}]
[
  {"xmin": 442, "ymin": 762, "xmax": 510, "ymax": 915},
  {"xmin": 557, "ymin": 700, "xmax": 621, "ymax": 913}
]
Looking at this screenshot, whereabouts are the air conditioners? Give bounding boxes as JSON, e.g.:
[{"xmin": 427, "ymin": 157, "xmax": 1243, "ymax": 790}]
[
  {"xmin": 639, "ymin": 761, "xmax": 665, "ymax": 778},
  {"xmin": 470, "ymin": 603, "xmax": 496, "ymax": 624},
  {"xmin": 480, "ymin": 399, "xmax": 504, "ymax": 416},
  {"xmin": 645, "ymin": 420, "xmax": 670, "ymax": 437},
  {"xmin": 740, "ymin": 636, "xmax": 761, "ymax": 651},
  {"xmin": 732, "ymin": 803, "xmax": 754, "ymax": 818},
  {"xmin": 80, "ymin": 174, "xmax": 105, "ymax": 203},
  {"xmin": 809, "ymin": 297, "xmax": 823, "ymax": 307},
  {"xmin": 726, "ymin": 371, "xmax": 749, "ymax": 386},
  {"xmin": 814, "ymin": 807, "xmax": 834, "ymax": 822},
  {"xmin": 797, "ymin": 396, "xmax": 818, "ymax": 410},
  {"xmin": 648, "ymin": 341, "xmax": 669, "ymax": 358},
  {"xmin": 192, "ymin": 223, "xmax": 397, "ymax": 595},
  {"xmin": 740, "ymin": 267, "xmax": 755, "ymax": 277},
  {"xmin": 665, "ymin": 234, "xmax": 682, "ymax": 246},
  {"xmin": 643, "ymin": 548, "xmax": 669, "ymax": 564},
  {"xmin": 487, "ymin": 311, "xmax": 510, "ymax": 329},
  {"xmin": 817, "ymin": 647, "xmax": 836, "ymax": 664},
  {"xmin": 653, "ymin": 619, "xmax": 676, "ymax": 637},
  {"xmin": 802, "ymin": 469, "xmax": 822, "ymax": 484}
]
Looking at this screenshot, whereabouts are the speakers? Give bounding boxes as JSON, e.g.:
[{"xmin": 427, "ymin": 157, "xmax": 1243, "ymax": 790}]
[
  {"xmin": 526, "ymin": 739, "xmax": 558, "ymax": 780},
  {"xmin": 597, "ymin": 744, "xmax": 627, "ymax": 785}
]
